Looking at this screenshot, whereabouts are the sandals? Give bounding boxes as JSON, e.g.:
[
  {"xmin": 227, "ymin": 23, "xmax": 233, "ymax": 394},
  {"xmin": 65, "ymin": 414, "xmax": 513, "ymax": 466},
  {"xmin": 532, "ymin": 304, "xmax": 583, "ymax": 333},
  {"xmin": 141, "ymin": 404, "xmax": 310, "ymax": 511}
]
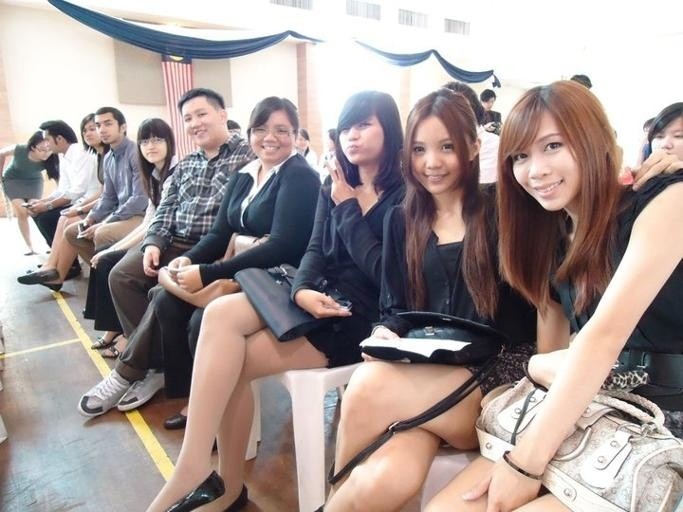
[{"xmin": 91, "ymin": 330, "xmax": 124, "ymax": 358}]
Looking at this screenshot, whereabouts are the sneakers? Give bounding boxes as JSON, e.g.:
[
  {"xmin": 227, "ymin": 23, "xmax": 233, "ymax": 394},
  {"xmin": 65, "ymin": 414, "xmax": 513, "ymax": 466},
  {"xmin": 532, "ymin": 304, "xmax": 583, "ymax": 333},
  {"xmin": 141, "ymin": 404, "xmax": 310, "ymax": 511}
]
[
  {"xmin": 77, "ymin": 368, "xmax": 130, "ymax": 417},
  {"xmin": 117, "ymin": 368, "xmax": 166, "ymax": 412}
]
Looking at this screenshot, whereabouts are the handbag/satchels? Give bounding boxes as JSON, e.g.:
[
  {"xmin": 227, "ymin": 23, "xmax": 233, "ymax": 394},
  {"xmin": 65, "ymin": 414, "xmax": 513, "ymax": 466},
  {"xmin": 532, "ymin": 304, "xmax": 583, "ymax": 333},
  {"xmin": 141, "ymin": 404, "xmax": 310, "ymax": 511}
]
[
  {"xmin": 234, "ymin": 263, "xmax": 355, "ymax": 342},
  {"xmin": 361, "ymin": 311, "xmax": 504, "ymax": 366},
  {"xmin": 474, "ymin": 377, "xmax": 683, "ymax": 511},
  {"xmin": 158, "ymin": 233, "xmax": 270, "ymax": 310}
]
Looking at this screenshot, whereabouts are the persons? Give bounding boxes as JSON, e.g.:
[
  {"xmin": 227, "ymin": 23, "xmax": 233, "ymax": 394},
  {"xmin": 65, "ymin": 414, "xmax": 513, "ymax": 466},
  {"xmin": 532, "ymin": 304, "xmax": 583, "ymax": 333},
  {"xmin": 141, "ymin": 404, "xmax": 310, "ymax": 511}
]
[{"xmin": 0, "ymin": 71, "xmax": 682, "ymax": 512}]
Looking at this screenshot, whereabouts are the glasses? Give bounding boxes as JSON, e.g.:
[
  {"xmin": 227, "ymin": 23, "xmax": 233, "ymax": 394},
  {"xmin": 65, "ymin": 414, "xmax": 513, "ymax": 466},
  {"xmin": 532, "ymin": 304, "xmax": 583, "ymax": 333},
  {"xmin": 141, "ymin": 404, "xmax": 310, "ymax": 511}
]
[
  {"xmin": 252, "ymin": 126, "xmax": 294, "ymax": 139},
  {"xmin": 137, "ymin": 138, "xmax": 165, "ymax": 145}
]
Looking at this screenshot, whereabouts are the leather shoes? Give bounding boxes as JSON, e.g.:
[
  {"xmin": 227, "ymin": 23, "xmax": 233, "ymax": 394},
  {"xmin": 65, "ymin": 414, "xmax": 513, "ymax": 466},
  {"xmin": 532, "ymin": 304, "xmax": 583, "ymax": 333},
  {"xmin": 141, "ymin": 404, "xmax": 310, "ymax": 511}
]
[
  {"xmin": 164, "ymin": 410, "xmax": 186, "ymax": 430},
  {"xmin": 166, "ymin": 470, "xmax": 247, "ymax": 511},
  {"xmin": 17, "ymin": 258, "xmax": 80, "ymax": 293}
]
